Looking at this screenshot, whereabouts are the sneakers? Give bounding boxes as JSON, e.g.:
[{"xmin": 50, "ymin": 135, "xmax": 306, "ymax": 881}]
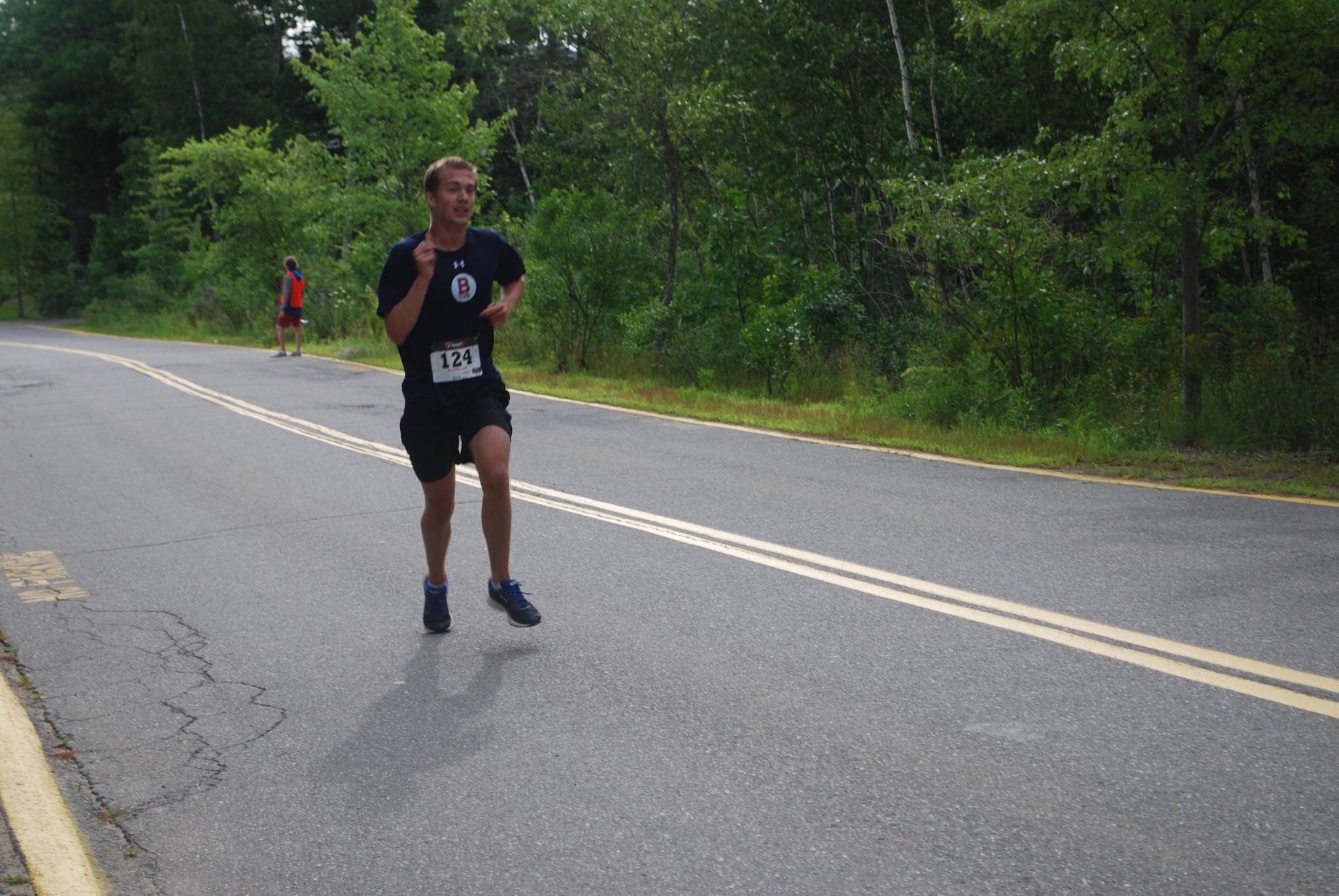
[
  {"xmin": 487, "ymin": 577, "xmax": 541, "ymax": 628},
  {"xmin": 272, "ymin": 352, "xmax": 287, "ymax": 357},
  {"xmin": 290, "ymin": 351, "xmax": 300, "ymax": 358},
  {"xmin": 422, "ymin": 577, "xmax": 451, "ymax": 633}
]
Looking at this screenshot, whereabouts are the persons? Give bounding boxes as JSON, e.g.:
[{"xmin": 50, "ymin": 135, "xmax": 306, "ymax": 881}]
[
  {"xmin": 376, "ymin": 155, "xmax": 543, "ymax": 634},
  {"xmin": 270, "ymin": 255, "xmax": 305, "ymax": 358}
]
